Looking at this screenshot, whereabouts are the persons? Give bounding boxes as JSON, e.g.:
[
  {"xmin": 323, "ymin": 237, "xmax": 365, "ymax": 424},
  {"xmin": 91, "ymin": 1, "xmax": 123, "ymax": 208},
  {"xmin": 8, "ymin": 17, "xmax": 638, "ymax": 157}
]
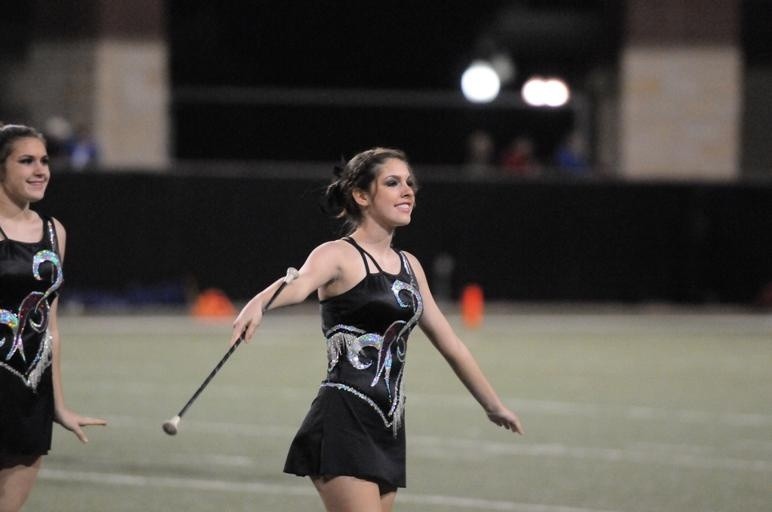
[
  {"xmin": 227, "ymin": 147, "xmax": 524, "ymax": 512},
  {"xmin": 0, "ymin": 124, "xmax": 108, "ymax": 512}
]
